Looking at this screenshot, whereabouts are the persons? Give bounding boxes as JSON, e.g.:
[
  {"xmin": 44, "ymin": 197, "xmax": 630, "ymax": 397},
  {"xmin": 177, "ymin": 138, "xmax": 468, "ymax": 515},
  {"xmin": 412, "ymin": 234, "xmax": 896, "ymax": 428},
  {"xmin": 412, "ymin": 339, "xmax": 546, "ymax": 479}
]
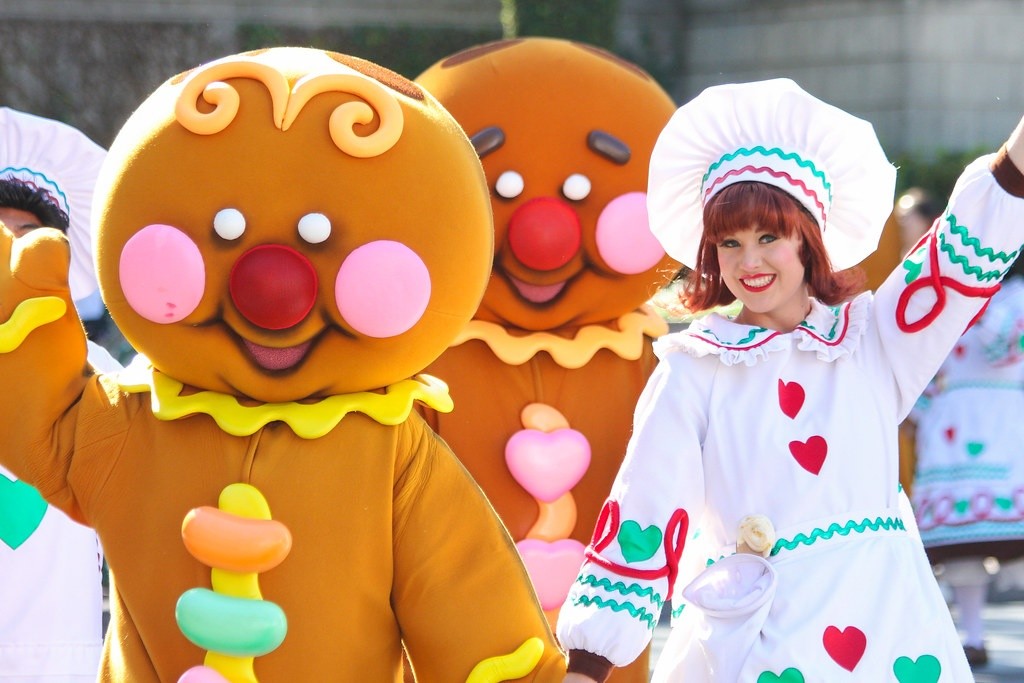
[
  {"xmin": 0, "ymin": 107, "xmax": 111, "ymax": 683},
  {"xmin": 904, "ymin": 168, "xmax": 1024, "ymax": 667},
  {"xmin": 554, "ymin": 79, "xmax": 1024, "ymax": 683}
]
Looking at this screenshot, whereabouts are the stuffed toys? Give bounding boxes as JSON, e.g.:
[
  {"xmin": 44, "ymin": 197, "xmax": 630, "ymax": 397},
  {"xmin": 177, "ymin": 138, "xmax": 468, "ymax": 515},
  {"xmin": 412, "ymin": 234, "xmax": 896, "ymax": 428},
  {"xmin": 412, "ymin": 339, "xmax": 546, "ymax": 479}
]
[
  {"xmin": 831, "ymin": 209, "xmax": 914, "ymax": 493},
  {"xmin": 0, "ymin": 43, "xmax": 571, "ymax": 683},
  {"xmin": 397, "ymin": 36, "xmax": 686, "ymax": 683}
]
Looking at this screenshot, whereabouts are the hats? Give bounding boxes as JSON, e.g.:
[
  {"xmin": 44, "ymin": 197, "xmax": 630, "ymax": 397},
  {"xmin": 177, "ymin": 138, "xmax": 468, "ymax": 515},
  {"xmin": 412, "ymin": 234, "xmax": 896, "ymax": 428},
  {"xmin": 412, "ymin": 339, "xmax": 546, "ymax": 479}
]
[
  {"xmin": 0, "ymin": 105, "xmax": 109, "ymax": 302},
  {"xmin": 646, "ymin": 78, "xmax": 900, "ymax": 283}
]
